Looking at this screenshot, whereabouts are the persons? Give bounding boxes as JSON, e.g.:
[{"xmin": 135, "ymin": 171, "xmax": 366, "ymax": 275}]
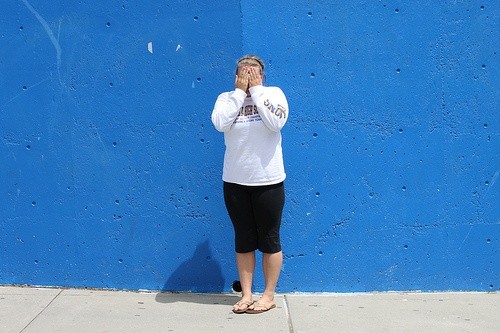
[{"xmin": 211, "ymin": 55, "xmax": 289, "ymax": 315}]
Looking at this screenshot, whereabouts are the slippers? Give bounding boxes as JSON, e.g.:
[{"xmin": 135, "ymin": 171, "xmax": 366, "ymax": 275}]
[{"xmin": 233, "ymin": 300, "xmax": 276, "ymax": 314}]
